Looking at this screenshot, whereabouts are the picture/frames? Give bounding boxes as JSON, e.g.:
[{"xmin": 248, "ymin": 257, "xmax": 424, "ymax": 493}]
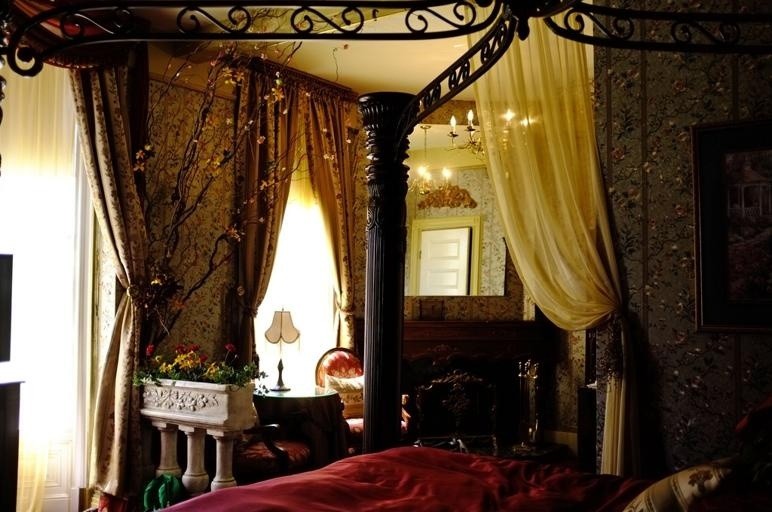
[{"xmin": 690, "ymin": 115, "xmax": 772, "ymax": 335}]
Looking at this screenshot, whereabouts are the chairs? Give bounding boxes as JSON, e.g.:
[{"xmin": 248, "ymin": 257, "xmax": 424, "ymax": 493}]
[
  {"xmin": 315, "ymin": 348, "xmax": 364, "ymax": 456},
  {"xmin": 234, "ymin": 413, "xmax": 315, "ymax": 484}
]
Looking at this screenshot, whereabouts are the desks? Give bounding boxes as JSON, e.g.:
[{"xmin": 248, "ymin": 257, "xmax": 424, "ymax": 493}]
[{"xmin": 253, "ymin": 384, "xmax": 350, "ymax": 466}]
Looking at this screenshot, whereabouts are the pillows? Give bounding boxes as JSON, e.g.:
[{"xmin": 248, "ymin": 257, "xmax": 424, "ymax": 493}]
[
  {"xmin": 621, "ymin": 456, "xmax": 736, "ymax": 512},
  {"xmin": 324, "ymin": 373, "xmax": 364, "ymax": 418}
]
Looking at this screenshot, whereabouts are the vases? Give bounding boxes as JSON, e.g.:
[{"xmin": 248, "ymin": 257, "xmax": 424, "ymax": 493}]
[{"xmin": 140, "ymin": 378, "xmax": 259, "ymax": 431}]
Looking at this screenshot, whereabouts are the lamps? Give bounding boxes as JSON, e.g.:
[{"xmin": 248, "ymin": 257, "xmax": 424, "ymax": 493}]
[
  {"xmin": 445, "ymin": 110, "xmax": 537, "ymax": 163},
  {"xmin": 264, "ymin": 309, "xmax": 300, "ymax": 391},
  {"xmin": 408, "ymin": 125, "xmax": 451, "ymax": 195}
]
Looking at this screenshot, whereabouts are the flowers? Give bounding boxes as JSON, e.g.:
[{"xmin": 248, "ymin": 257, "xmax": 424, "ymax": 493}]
[{"xmin": 133, "ymin": 341, "xmax": 268, "ymax": 387}]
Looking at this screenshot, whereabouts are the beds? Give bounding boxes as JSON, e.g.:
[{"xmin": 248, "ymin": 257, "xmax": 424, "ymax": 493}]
[{"xmin": 157, "ymin": 388, "xmax": 771, "ymax": 512}]
